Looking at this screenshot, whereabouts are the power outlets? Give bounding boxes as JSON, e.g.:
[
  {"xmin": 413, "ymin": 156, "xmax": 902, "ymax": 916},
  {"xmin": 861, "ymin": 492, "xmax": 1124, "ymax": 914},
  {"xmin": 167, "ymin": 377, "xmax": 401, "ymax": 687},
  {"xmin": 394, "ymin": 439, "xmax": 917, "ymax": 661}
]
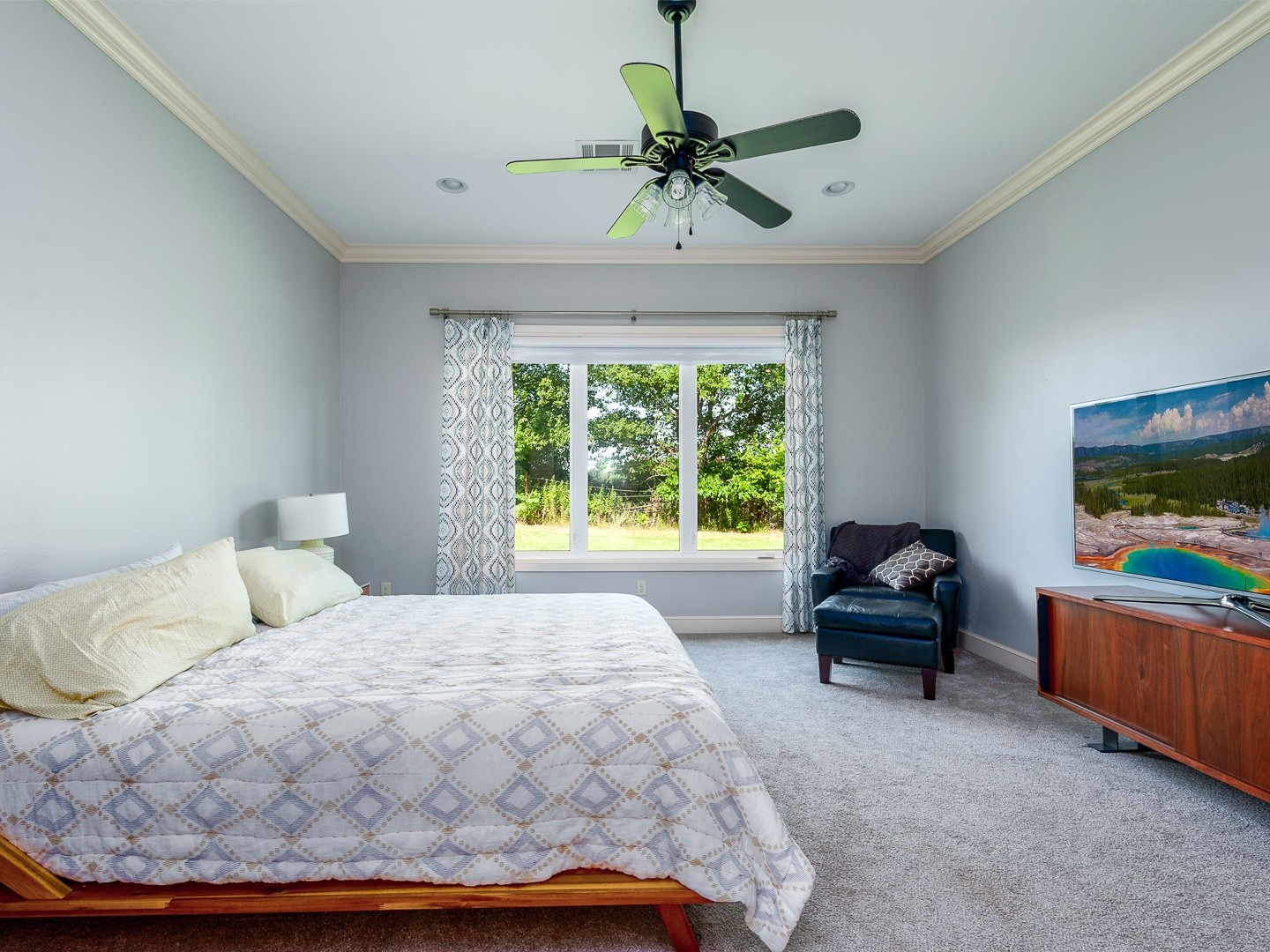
[
  {"xmin": 380, "ymin": 583, "xmax": 391, "ymax": 596},
  {"xmin": 637, "ymin": 581, "xmax": 646, "ymax": 596}
]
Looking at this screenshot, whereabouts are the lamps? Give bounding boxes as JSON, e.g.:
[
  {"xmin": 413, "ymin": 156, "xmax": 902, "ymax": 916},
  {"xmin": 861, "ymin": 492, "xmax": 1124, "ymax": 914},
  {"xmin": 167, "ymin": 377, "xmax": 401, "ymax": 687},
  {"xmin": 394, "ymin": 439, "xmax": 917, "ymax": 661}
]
[
  {"xmin": 277, "ymin": 492, "xmax": 350, "ymax": 564},
  {"xmin": 631, "ymin": 157, "xmax": 728, "ymax": 249}
]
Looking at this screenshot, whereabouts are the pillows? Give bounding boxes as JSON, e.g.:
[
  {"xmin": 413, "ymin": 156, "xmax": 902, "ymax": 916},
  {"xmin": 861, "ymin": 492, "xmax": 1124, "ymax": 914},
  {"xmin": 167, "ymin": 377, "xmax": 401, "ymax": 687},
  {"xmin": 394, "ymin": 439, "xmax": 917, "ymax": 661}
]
[
  {"xmin": 868, "ymin": 540, "xmax": 957, "ymax": 590},
  {"xmin": 0, "ymin": 537, "xmax": 363, "ymax": 720}
]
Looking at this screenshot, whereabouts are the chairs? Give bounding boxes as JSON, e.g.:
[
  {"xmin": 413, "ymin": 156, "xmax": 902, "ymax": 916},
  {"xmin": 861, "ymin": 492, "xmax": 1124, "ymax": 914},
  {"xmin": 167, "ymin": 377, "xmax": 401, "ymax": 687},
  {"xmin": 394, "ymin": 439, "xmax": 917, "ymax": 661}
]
[{"xmin": 811, "ymin": 527, "xmax": 963, "ymax": 701}]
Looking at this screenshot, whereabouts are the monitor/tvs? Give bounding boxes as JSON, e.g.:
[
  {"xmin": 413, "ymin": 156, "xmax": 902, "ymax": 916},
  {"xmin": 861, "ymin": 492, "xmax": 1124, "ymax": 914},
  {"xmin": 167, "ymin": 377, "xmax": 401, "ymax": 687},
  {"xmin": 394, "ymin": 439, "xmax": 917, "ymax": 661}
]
[{"xmin": 1070, "ymin": 370, "xmax": 1270, "ymax": 630}]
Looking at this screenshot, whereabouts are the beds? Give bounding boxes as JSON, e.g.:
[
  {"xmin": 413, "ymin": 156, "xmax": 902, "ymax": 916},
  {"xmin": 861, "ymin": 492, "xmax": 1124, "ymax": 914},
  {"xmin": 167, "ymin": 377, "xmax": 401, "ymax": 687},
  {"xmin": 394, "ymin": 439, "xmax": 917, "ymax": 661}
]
[{"xmin": 0, "ymin": 538, "xmax": 817, "ymax": 952}]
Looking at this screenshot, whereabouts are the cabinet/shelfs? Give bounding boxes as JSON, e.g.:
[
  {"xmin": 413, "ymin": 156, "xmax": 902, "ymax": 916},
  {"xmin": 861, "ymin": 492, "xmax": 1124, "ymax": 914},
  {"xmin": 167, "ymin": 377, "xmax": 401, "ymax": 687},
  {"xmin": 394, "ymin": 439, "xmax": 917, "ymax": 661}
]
[{"xmin": 1035, "ymin": 585, "xmax": 1270, "ymax": 803}]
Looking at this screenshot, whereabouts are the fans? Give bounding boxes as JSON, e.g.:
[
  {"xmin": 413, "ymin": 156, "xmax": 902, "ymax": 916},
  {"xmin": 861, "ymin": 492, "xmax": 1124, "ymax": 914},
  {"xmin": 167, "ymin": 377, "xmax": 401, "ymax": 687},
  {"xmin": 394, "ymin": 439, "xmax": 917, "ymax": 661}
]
[{"xmin": 507, "ymin": 0, "xmax": 860, "ymax": 238}]
